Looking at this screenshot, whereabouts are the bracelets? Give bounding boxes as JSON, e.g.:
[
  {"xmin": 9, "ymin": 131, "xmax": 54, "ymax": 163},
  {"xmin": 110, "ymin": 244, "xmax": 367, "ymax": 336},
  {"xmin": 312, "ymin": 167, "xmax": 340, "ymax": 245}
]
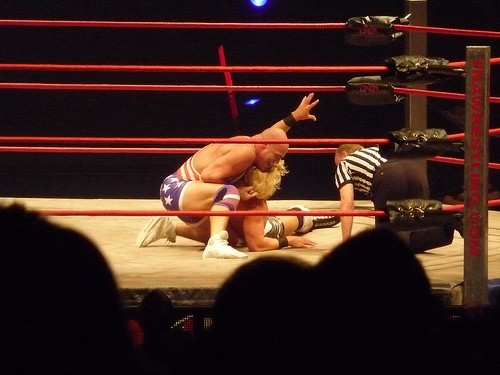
[
  {"xmin": 283, "ymin": 114, "xmax": 297, "ymax": 126},
  {"xmin": 277, "ymin": 237, "xmax": 289, "ymax": 249}
]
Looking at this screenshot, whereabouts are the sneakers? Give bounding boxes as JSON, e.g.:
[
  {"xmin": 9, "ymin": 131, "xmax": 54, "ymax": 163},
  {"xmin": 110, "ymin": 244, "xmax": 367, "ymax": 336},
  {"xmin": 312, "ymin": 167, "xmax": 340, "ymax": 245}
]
[
  {"xmin": 137, "ymin": 215, "xmax": 176, "ymax": 247},
  {"xmin": 202, "ymin": 238, "xmax": 248, "ymax": 259},
  {"xmin": 312, "ymin": 216, "xmax": 340, "ymax": 231}
]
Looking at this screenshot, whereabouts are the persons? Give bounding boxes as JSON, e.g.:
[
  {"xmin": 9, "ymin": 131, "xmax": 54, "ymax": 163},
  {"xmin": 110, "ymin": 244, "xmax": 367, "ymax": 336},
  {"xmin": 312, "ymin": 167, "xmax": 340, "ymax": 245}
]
[
  {"xmin": 0, "ymin": 198, "xmax": 500, "ymax": 375},
  {"xmin": 138, "ymin": 92, "xmax": 468, "ymax": 252}
]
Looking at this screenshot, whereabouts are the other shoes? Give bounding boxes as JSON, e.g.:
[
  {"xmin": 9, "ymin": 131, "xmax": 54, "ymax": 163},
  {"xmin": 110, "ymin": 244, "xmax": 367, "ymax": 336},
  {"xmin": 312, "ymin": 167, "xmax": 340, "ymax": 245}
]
[{"xmin": 452, "ymin": 213, "xmax": 463, "ymax": 238}]
